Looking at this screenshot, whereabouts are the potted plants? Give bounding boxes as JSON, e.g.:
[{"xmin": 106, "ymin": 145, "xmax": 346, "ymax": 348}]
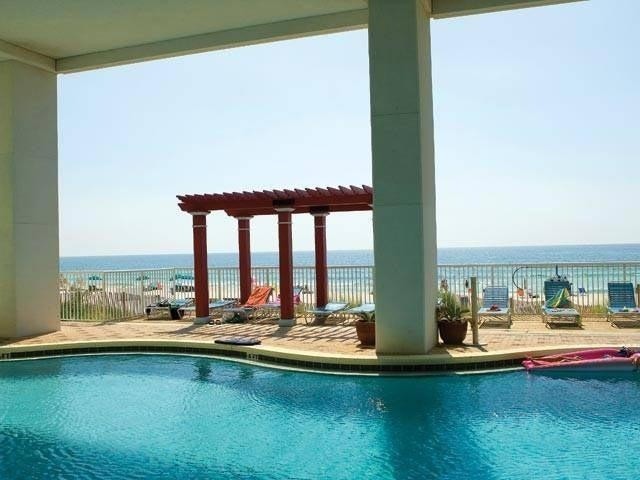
[
  {"xmin": 355, "ymin": 310, "xmax": 375, "ymax": 346},
  {"xmin": 435, "ymin": 278, "xmax": 471, "ymax": 345}
]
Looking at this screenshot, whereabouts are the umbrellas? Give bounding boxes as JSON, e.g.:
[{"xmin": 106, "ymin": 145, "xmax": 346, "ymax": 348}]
[
  {"xmin": 89, "ymin": 276, "xmax": 102, "ymax": 280},
  {"xmin": 181, "ymin": 274, "xmax": 195, "ymax": 279},
  {"xmin": 136, "ymin": 277, "xmax": 150, "ymax": 280},
  {"xmin": 169, "ymin": 273, "xmax": 181, "ymax": 282}
]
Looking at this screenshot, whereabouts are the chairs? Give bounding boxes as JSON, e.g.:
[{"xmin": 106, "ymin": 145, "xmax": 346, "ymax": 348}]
[
  {"xmin": 477, "ymin": 286, "xmax": 513, "ymax": 329},
  {"xmin": 541, "ymin": 280, "xmax": 582, "ymax": 330},
  {"xmin": 607, "ymin": 282, "xmax": 640, "ymax": 330},
  {"xmin": 304, "ymin": 301, "xmax": 376, "ymax": 326},
  {"xmin": 145, "ymin": 284, "xmax": 303, "ymax": 319},
  {"xmin": 88, "ymin": 285, "xmax": 103, "ymax": 292},
  {"xmin": 141, "ymin": 284, "xmax": 162, "ymax": 294}
]
